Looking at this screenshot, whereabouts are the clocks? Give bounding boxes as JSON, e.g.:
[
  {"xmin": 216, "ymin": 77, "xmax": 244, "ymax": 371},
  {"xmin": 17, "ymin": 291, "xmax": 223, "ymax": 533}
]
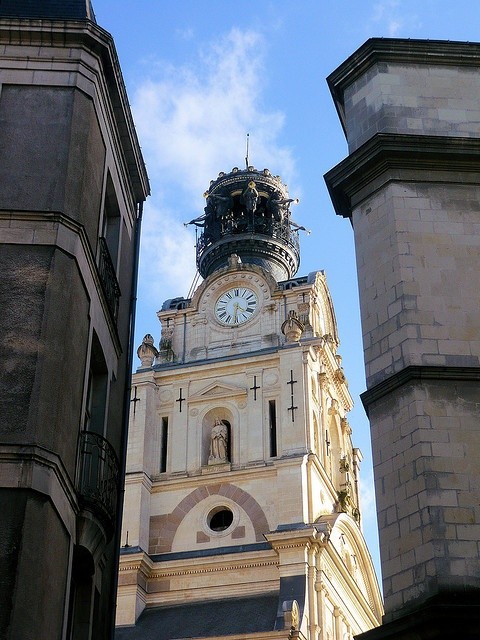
[{"xmin": 207, "ymin": 280, "xmax": 262, "ymax": 330}]
